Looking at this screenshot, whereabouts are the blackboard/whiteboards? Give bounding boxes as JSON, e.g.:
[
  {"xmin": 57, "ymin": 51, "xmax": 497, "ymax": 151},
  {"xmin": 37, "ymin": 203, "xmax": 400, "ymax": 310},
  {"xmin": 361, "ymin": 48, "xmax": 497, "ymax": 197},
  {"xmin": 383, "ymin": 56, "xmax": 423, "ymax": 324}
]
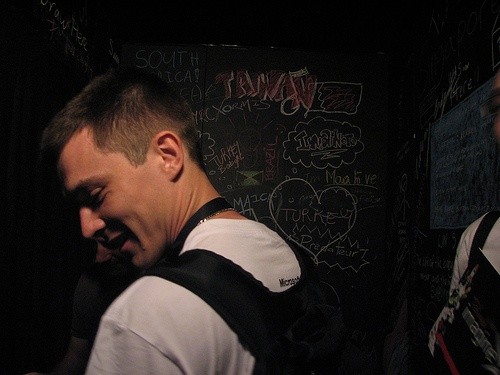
[{"xmin": 0, "ymin": 0, "xmax": 500, "ymax": 375}]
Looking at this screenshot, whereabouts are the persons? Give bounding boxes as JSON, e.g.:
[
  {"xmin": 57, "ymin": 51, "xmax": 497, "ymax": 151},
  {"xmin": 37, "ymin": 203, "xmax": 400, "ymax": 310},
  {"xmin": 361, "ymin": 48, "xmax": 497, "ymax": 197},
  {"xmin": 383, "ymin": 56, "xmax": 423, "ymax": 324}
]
[
  {"xmin": 427, "ymin": 67, "xmax": 500, "ymax": 375},
  {"xmin": 25, "ymin": 65, "xmax": 318, "ymax": 375}
]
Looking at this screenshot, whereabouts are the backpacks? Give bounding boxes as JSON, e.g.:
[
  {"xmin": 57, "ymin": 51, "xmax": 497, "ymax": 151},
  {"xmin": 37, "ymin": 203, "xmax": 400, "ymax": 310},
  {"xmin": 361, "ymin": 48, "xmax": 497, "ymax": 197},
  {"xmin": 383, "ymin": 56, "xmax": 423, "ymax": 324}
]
[{"xmin": 137, "ymin": 226, "xmax": 383, "ymax": 375}]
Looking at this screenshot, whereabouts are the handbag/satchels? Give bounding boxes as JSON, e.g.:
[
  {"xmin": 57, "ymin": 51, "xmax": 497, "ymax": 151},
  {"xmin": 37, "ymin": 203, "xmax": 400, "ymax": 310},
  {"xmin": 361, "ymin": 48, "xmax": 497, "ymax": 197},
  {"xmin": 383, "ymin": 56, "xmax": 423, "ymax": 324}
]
[{"xmin": 432, "ymin": 248, "xmax": 500, "ymax": 375}]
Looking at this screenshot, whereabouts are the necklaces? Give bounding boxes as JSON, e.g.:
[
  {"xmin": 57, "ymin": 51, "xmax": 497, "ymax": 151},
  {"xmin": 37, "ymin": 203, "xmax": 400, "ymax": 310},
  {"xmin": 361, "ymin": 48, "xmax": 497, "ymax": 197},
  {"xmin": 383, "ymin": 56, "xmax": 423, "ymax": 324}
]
[{"xmin": 196, "ymin": 207, "xmax": 238, "ymax": 227}]
